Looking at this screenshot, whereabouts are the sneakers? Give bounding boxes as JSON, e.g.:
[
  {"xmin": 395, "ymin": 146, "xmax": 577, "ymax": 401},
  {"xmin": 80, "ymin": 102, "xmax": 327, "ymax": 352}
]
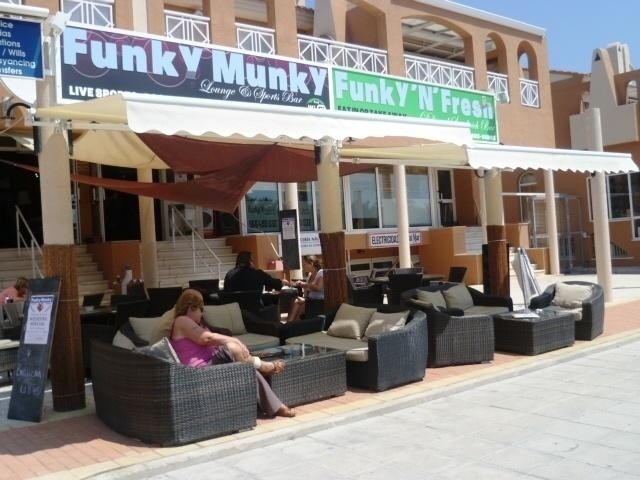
[
  {"xmin": 259, "ymin": 359, "xmax": 287, "ymax": 377},
  {"xmin": 264, "ymin": 405, "xmax": 296, "ymax": 417}
]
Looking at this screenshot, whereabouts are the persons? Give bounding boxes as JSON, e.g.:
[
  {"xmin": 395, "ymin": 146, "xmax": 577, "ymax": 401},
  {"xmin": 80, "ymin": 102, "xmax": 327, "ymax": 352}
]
[
  {"xmin": 170, "ymin": 293, "xmax": 297, "ymax": 418},
  {"xmin": 0, "ymin": 277, "xmax": 29, "ymax": 305},
  {"xmin": 150, "ymin": 288, "xmax": 203, "ymax": 346},
  {"xmin": 223, "ymin": 251, "xmax": 290, "ymax": 319},
  {"xmin": 287, "ymin": 255, "xmax": 324, "ymax": 321}
]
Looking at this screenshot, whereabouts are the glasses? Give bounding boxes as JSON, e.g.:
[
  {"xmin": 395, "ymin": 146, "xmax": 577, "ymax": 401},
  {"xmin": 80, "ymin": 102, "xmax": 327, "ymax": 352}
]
[{"xmin": 195, "ymin": 304, "xmax": 204, "ymax": 312}]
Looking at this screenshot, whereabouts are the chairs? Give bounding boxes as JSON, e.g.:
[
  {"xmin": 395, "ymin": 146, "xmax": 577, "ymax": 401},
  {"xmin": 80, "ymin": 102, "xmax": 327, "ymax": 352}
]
[
  {"xmin": 528, "ymin": 281, "xmax": 604, "ymax": 341},
  {"xmin": 0, "ymin": 338, "xmax": 20, "ymax": 377},
  {"xmin": 189, "ymin": 279, "xmax": 220, "ymax": 305},
  {"xmin": 82, "ymin": 293, "xmax": 104, "ymax": 307},
  {"xmin": 345, "ymin": 266, "xmax": 467, "ymax": 306}
]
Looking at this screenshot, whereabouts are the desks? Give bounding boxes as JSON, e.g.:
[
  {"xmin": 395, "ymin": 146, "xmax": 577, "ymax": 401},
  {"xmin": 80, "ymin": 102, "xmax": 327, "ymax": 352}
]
[{"xmin": 79, "ymin": 305, "xmax": 118, "ymax": 378}]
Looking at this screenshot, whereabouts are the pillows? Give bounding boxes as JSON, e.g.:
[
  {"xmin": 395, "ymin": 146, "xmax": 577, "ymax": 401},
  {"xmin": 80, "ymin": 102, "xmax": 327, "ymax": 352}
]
[{"xmin": 548, "ymin": 281, "xmax": 595, "ymax": 310}]
